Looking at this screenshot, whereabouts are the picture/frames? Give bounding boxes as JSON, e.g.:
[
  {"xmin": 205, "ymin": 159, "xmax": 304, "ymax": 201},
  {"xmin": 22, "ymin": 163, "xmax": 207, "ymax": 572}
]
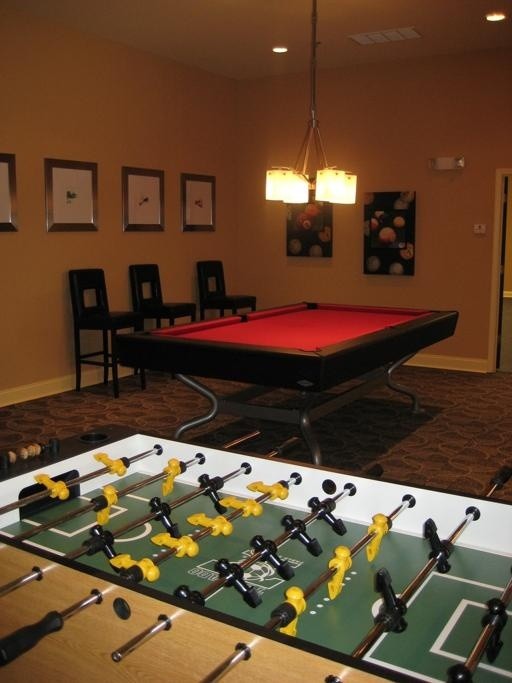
[{"xmin": 0, "ymin": 153, "xmax": 217, "ymax": 233}]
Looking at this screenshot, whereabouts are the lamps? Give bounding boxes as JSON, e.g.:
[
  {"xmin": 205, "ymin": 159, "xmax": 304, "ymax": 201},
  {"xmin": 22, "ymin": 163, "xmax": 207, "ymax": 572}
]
[{"xmin": 265, "ymin": 1, "xmax": 358, "ymax": 205}]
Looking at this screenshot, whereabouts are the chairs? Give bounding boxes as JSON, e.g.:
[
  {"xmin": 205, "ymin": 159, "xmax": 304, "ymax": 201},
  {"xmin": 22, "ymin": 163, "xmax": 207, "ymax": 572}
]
[{"xmin": 67, "ymin": 259, "xmax": 257, "ymax": 400}]
